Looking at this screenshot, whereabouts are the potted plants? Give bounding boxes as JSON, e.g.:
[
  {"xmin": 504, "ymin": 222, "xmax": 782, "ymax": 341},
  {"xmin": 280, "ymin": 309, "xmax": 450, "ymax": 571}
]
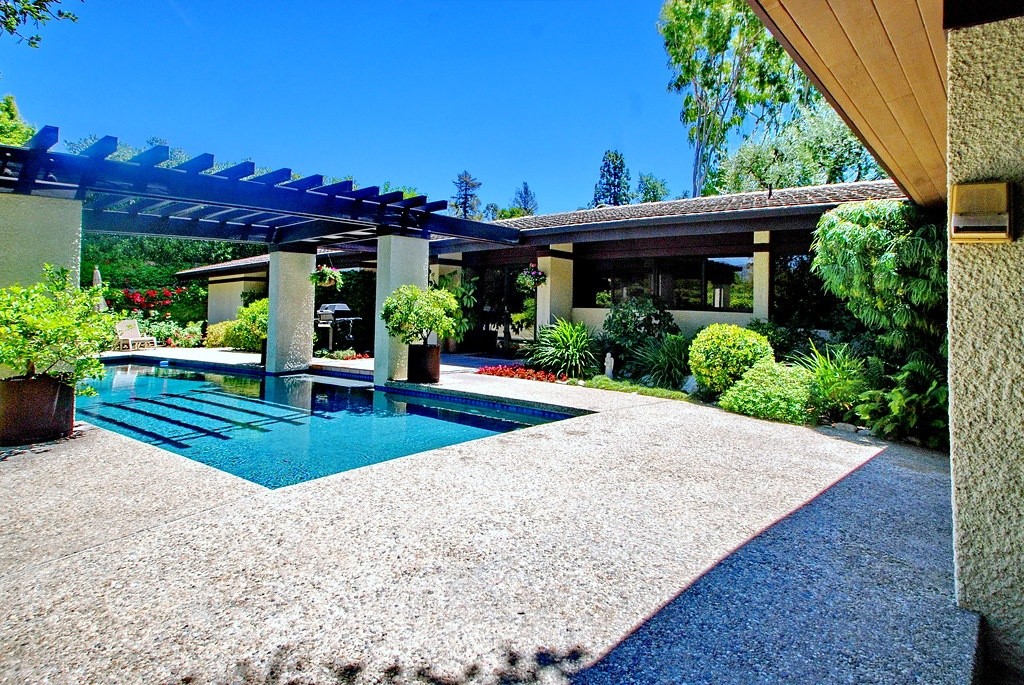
[
  {"xmin": 0, "ymin": 263, "xmax": 136, "ymax": 447},
  {"xmin": 438, "ymin": 269, "xmax": 479, "ymax": 353},
  {"xmin": 473, "ymin": 281, "xmax": 505, "ymax": 353},
  {"xmin": 378, "ymin": 283, "xmax": 460, "ymax": 384}
]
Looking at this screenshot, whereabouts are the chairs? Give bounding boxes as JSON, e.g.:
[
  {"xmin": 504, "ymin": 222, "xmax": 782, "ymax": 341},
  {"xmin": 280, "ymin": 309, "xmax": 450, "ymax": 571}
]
[{"xmin": 116, "ymin": 319, "xmax": 157, "ymax": 352}]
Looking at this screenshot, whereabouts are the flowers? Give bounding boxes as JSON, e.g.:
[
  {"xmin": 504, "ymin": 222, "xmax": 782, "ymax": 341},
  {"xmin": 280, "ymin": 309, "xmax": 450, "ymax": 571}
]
[
  {"xmin": 306, "ymin": 263, "xmax": 344, "ymax": 292},
  {"xmin": 514, "ymin": 262, "xmax": 547, "ymax": 292}
]
